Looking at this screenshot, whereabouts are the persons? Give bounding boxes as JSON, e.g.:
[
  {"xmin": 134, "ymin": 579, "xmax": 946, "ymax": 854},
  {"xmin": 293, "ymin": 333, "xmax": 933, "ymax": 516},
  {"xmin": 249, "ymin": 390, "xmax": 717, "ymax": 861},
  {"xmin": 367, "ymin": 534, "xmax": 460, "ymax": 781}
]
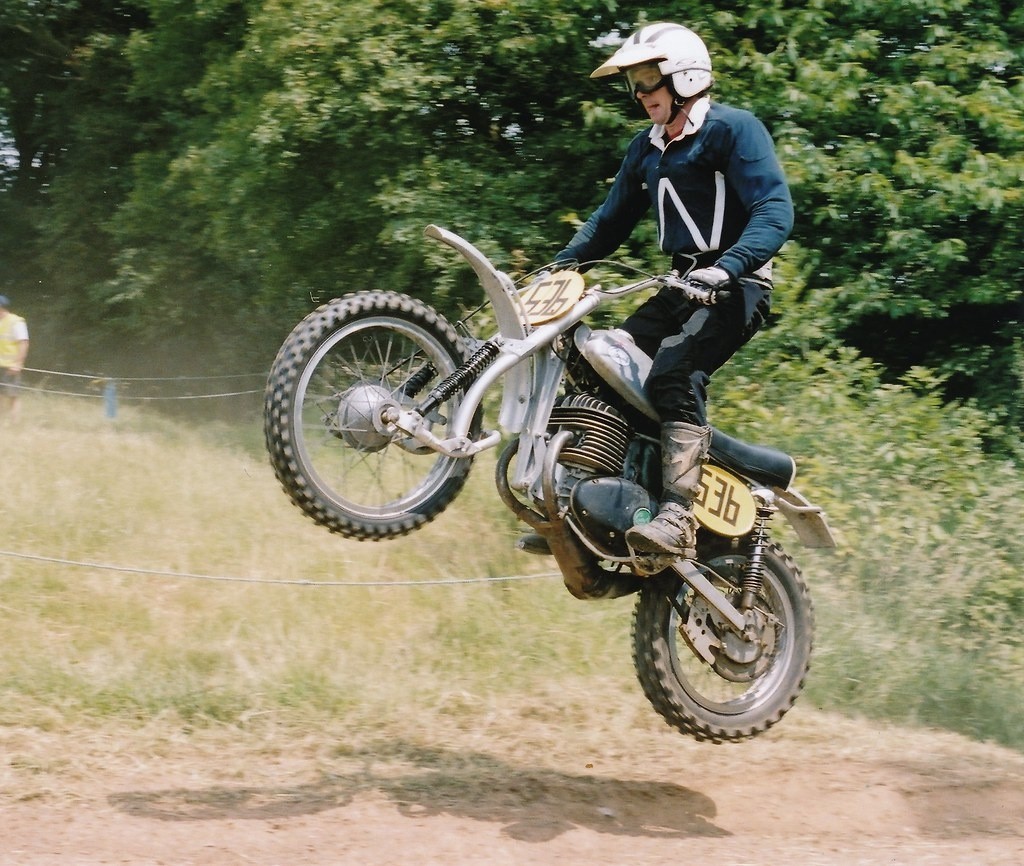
[
  {"xmin": 0, "ymin": 295, "xmax": 29, "ymax": 420},
  {"xmin": 515, "ymin": 21, "xmax": 793, "ymax": 560}
]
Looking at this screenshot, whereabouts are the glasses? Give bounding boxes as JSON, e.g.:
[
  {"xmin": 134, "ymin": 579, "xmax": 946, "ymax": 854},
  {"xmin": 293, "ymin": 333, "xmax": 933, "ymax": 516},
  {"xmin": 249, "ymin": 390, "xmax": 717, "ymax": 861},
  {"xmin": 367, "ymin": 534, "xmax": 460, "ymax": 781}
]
[{"xmin": 623, "ymin": 60, "xmax": 669, "ymax": 100}]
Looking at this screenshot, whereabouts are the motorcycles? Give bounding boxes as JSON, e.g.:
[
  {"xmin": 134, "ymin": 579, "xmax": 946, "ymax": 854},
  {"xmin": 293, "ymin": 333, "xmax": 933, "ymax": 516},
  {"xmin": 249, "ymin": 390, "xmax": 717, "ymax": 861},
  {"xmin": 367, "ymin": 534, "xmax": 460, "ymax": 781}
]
[{"xmin": 264, "ymin": 221, "xmax": 848, "ymax": 753}]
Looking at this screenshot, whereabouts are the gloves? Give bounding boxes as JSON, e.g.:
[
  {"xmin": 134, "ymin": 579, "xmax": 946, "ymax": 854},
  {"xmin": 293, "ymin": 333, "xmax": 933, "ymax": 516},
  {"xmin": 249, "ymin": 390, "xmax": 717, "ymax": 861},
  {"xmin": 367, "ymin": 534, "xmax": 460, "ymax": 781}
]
[{"xmin": 683, "ymin": 266, "xmax": 731, "ymax": 306}]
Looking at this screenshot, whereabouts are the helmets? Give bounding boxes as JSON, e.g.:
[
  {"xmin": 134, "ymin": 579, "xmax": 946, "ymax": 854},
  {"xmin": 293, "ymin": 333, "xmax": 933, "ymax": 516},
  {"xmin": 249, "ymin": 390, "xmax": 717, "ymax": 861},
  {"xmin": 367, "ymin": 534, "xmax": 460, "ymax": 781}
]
[{"xmin": 589, "ymin": 23, "xmax": 713, "ymax": 98}]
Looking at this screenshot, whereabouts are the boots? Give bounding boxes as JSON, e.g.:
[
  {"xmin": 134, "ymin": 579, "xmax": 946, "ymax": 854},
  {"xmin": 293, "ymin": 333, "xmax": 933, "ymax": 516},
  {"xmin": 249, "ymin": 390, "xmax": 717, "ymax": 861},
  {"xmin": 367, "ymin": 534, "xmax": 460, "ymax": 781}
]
[
  {"xmin": 519, "ymin": 534, "xmax": 553, "ymax": 556},
  {"xmin": 624, "ymin": 421, "xmax": 713, "ymax": 559}
]
